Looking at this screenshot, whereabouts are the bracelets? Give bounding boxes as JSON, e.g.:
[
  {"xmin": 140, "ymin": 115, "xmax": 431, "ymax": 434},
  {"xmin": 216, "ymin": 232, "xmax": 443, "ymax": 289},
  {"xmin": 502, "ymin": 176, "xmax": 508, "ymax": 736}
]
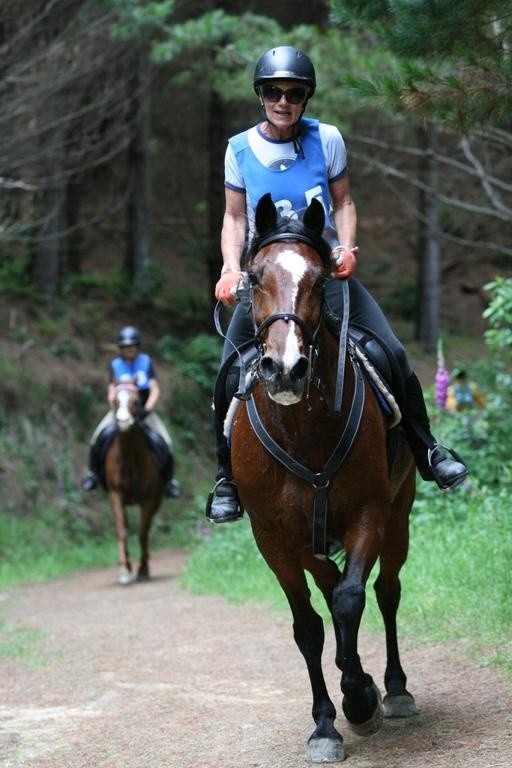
[{"xmin": 140, "ymin": 408, "xmax": 150, "ymax": 420}]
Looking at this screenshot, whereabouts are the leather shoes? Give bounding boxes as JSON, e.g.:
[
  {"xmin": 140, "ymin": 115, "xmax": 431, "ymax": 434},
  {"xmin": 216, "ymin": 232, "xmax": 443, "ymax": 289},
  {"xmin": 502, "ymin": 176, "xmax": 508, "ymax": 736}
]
[
  {"xmin": 82, "ymin": 473, "xmax": 97, "ymax": 491},
  {"xmin": 419, "ymin": 453, "xmax": 469, "ymax": 485},
  {"xmin": 166, "ymin": 484, "xmax": 179, "ymax": 498},
  {"xmin": 209, "ymin": 484, "xmax": 238, "ymax": 519}
]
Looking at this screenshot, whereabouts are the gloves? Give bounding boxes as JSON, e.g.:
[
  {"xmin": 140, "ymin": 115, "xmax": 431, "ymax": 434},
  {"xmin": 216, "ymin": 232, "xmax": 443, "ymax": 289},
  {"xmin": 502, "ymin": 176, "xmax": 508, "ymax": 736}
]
[
  {"xmin": 215, "ymin": 271, "xmax": 249, "ymax": 309},
  {"xmin": 329, "ymin": 248, "xmax": 357, "ymax": 281}
]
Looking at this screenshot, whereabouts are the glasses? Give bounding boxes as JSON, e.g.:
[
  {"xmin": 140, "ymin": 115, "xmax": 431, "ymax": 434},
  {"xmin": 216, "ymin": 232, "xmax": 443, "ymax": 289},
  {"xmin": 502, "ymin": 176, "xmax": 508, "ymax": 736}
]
[{"xmin": 258, "ymin": 84, "xmax": 311, "ymax": 104}]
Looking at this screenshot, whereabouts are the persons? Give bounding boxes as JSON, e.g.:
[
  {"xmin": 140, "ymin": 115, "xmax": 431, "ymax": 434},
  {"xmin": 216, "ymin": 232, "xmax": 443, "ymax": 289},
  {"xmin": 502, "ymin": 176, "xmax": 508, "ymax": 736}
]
[
  {"xmin": 82, "ymin": 325, "xmax": 181, "ymax": 500},
  {"xmin": 445, "ymin": 367, "xmax": 487, "ymax": 413},
  {"xmin": 206, "ymin": 46, "xmax": 470, "ymax": 524}
]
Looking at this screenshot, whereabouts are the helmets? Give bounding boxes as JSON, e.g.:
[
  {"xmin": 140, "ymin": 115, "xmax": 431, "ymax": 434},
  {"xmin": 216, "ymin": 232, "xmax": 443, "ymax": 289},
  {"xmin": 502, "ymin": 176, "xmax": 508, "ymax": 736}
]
[
  {"xmin": 253, "ymin": 45, "xmax": 315, "ymax": 99},
  {"xmin": 116, "ymin": 325, "xmax": 141, "ymax": 347}
]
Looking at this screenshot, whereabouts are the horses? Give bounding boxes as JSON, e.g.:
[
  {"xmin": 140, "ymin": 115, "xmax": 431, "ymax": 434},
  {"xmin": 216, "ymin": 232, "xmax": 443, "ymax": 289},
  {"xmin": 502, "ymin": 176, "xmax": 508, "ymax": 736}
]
[
  {"xmin": 230, "ymin": 191, "xmax": 416, "ymax": 763},
  {"xmin": 96, "ymin": 377, "xmax": 175, "ymax": 586}
]
